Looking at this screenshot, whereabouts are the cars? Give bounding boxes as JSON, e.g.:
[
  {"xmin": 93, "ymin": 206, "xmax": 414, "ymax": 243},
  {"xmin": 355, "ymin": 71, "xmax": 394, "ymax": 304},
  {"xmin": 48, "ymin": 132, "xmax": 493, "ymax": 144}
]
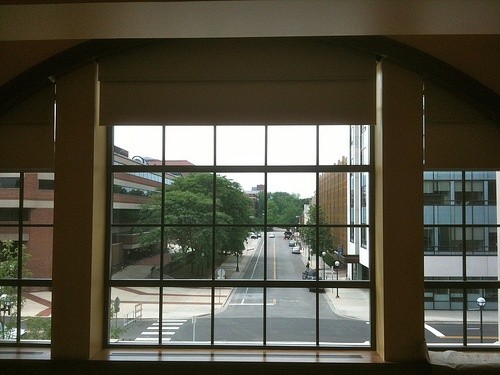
[
  {"xmin": 250, "ymin": 231, "xmax": 261, "ymax": 239},
  {"xmin": 289, "ymin": 240, "xmax": 295, "ymax": 247},
  {"xmin": 302, "ymin": 271, "xmax": 324, "ymax": 293},
  {"xmin": 292, "ymin": 247, "xmax": 300, "ymax": 254},
  {"xmin": 269, "ymin": 233, "xmax": 275, "ymax": 238}
]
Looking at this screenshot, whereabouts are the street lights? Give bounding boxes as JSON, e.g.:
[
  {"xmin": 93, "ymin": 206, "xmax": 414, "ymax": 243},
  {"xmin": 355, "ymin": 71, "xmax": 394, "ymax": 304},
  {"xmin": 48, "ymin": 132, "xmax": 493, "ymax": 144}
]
[
  {"xmin": 476, "ymin": 297, "xmax": 486, "ymax": 344},
  {"xmin": 321, "ymin": 251, "xmax": 327, "ymax": 279},
  {"xmin": 334, "ymin": 260, "xmax": 340, "ymax": 297}
]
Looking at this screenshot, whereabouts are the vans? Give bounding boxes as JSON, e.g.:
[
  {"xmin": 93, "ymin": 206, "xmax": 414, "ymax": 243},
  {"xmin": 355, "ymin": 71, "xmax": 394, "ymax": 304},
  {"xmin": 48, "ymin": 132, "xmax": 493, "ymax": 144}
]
[{"xmin": 284, "ymin": 231, "xmax": 292, "ymax": 240}]
